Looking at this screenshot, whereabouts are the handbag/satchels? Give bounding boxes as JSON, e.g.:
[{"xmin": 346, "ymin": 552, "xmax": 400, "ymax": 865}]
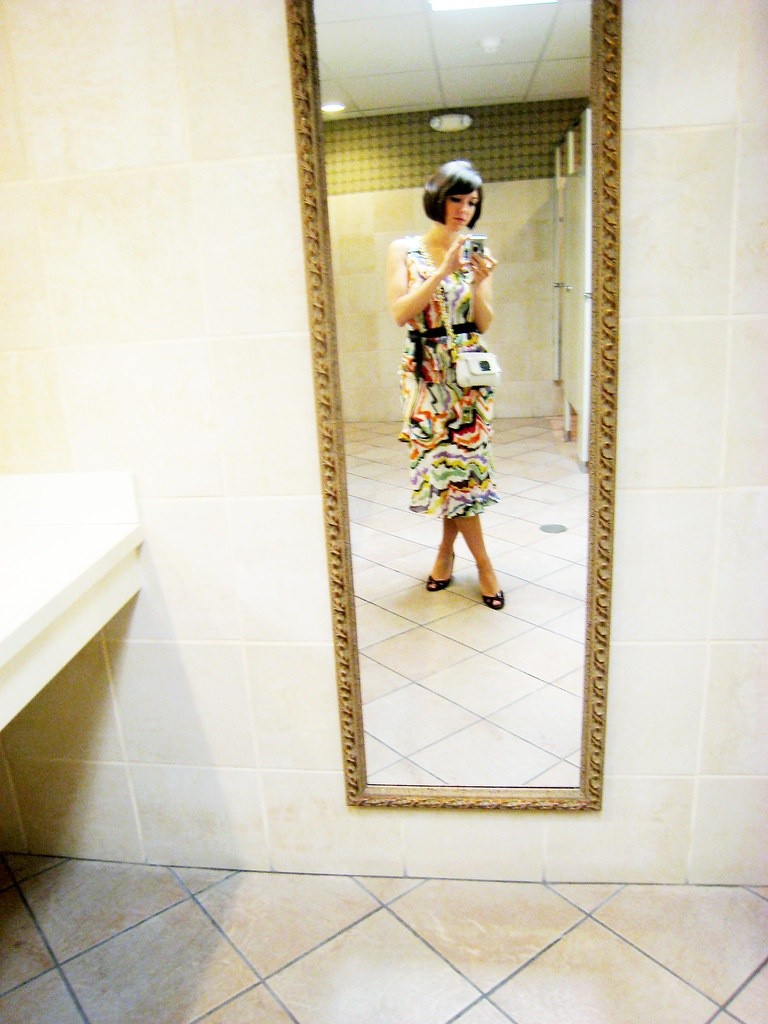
[{"xmin": 456, "ymin": 353, "xmax": 498, "ymax": 387}]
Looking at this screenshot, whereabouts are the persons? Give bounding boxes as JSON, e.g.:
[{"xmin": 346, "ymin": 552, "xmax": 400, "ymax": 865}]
[{"xmin": 386, "ymin": 161, "xmax": 504, "ymax": 610}]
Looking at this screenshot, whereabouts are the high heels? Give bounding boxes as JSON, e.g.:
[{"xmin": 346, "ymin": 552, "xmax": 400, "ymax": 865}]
[
  {"xmin": 482, "ymin": 589, "xmax": 504, "ymax": 610},
  {"xmin": 426, "ymin": 552, "xmax": 455, "ymax": 591}
]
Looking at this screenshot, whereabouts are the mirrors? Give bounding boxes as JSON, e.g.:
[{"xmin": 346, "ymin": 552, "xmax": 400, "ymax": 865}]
[{"xmin": 286, "ymin": 0, "xmax": 624, "ymax": 816}]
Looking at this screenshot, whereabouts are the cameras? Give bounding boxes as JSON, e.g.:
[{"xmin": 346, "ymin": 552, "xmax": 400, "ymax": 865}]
[{"xmin": 470, "ymin": 237, "xmax": 485, "ymax": 258}]
